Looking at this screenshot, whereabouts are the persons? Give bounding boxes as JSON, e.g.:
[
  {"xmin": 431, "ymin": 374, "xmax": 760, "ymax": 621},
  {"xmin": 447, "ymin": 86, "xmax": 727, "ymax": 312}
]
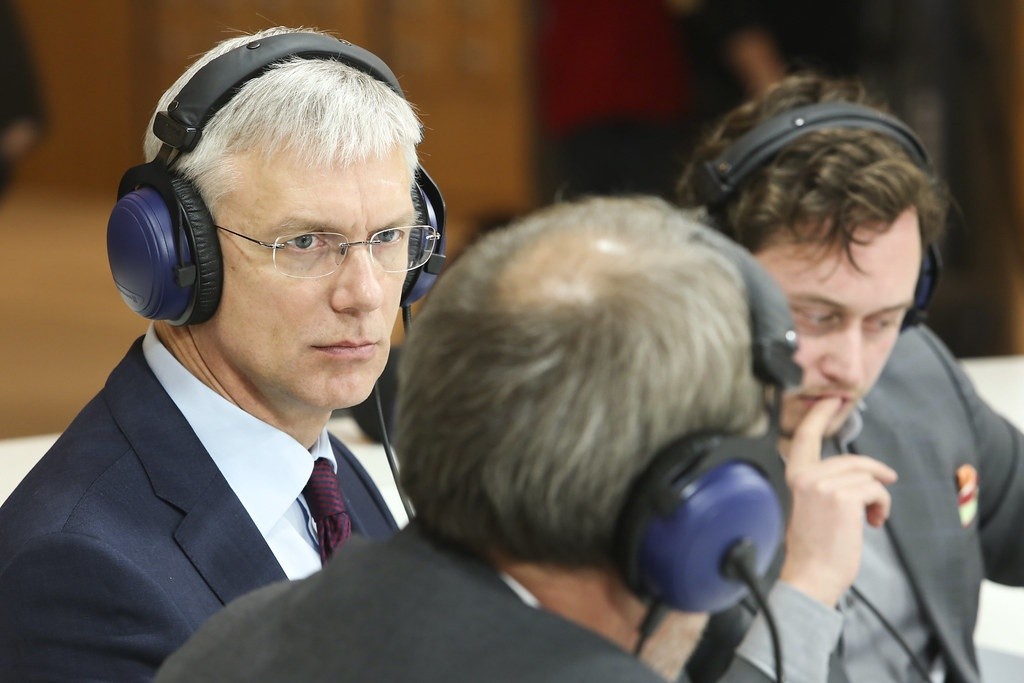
[
  {"xmin": 0, "ymin": 0, "xmax": 47, "ymax": 198},
  {"xmin": 150, "ymin": 196, "xmax": 768, "ymax": 682},
  {"xmin": 0, "ymin": 27, "xmax": 421, "ymax": 682},
  {"xmin": 664, "ymin": 0, "xmax": 790, "ymax": 115},
  {"xmin": 674, "ymin": 69, "xmax": 1024, "ymax": 683}
]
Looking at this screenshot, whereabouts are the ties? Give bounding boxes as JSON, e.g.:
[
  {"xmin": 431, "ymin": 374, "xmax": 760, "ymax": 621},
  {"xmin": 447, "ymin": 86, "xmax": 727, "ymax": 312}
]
[{"xmin": 301, "ymin": 456, "xmax": 352, "ymax": 569}]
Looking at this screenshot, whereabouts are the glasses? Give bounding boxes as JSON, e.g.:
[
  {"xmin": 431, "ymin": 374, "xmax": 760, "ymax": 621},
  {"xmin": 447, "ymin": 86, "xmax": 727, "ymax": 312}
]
[{"xmin": 214, "ymin": 225, "xmax": 441, "ymax": 279}]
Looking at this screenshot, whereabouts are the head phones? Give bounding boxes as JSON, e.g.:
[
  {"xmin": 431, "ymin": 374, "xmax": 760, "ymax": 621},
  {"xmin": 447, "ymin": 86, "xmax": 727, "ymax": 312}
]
[
  {"xmin": 106, "ymin": 34, "xmax": 448, "ymax": 327},
  {"xmin": 685, "ymin": 99, "xmax": 940, "ymax": 334},
  {"xmin": 614, "ymin": 223, "xmax": 804, "ymax": 616}
]
